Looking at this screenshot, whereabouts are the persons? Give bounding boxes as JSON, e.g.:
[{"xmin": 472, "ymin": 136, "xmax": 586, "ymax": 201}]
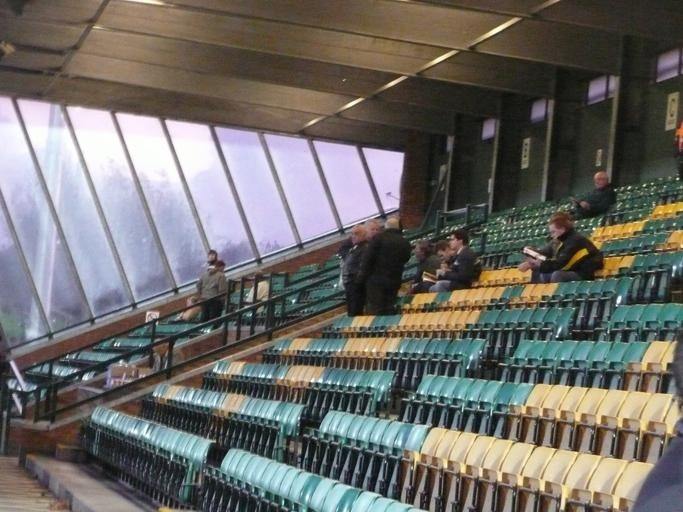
[
  {"xmin": 519, "ymin": 212, "xmax": 604, "ymax": 284},
  {"xmin": 151, "ymin": 338, "xmax": 184, "ymax": 372},
  {"xmin": 569, "ymin": 172, "xmax": 617, "ymax": 220},
  {"xmin": 174, "ymin": 250, "xmax": 269, "ymax": 321},
  {"xmin": 335, "ymin": 213, "xmax": 483, "ymax": 316},
  {"xmin": 674, "ymin": 120, "xmax": 683, "ymax": 181}
]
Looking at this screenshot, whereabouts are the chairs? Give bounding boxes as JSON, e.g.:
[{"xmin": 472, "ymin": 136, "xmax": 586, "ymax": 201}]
[{"xmin": 79, "ymin": 173, "xmax": 683, "ymax": 512}]
